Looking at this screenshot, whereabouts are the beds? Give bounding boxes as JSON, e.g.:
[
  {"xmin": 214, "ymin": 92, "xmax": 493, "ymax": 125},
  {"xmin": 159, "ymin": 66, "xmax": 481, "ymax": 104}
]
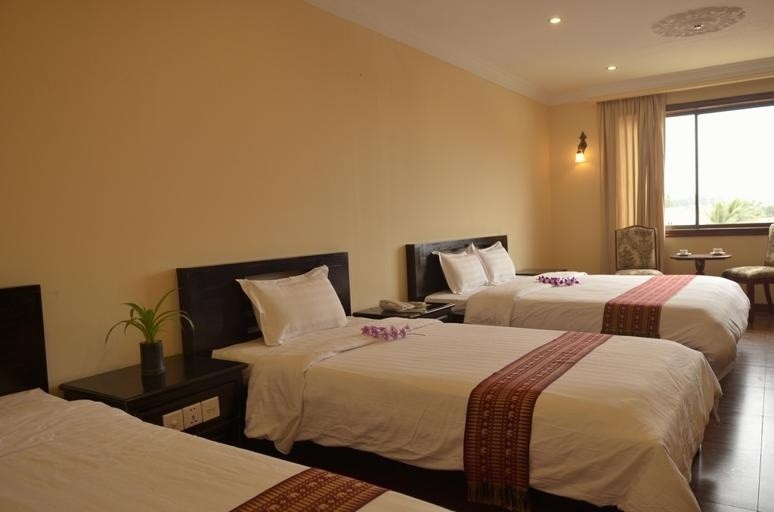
[
  {"xmin": 405, "ymin": 235, "xmax": 751, "ymax": 381},
  {"xmin": 0, "ymin": 283, "xmax": 451, "ymax": 512},
  {"xmin": 175, "ymin": 253, "xmax": 723, "ymax": 512}
]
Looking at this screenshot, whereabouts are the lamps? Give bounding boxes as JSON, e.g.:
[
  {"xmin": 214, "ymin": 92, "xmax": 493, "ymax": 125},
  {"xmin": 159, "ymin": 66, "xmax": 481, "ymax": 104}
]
[{"xmin": 575, "ymin": 132, "xmax": 587, "ymax": 163}]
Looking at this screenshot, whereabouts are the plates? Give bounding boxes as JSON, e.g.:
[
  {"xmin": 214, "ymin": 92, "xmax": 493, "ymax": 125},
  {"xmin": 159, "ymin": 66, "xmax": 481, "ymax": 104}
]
[
  {"xmin": 710, "ymin": 252, "xmax": 725, "ymax": 255},
  {"xmin": 676, "ymin": 253, "xmax": 692, "ymax": 256}
]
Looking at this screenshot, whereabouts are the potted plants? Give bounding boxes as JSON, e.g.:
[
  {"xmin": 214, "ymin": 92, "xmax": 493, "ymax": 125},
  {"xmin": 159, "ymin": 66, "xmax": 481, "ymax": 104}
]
[{"xmin": 104, "ymin": 289, "xmax": 196, "ymax": 375}]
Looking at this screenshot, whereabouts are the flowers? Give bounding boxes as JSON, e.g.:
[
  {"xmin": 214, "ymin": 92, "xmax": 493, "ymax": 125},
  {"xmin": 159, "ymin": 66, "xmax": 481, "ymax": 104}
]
[
  {"xmin": 536, "ymin": 274, "xmax": 579, "ymax": 286},
  {"xmin": 362, "ymin": 323, "xmax": 425, "ymax": 341}
]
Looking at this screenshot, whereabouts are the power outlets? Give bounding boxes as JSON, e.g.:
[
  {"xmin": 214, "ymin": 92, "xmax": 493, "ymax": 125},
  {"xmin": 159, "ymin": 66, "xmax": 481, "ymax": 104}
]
[{"xmin": 183, "ymin": 404, "xmax": 203, "ymax": 428}]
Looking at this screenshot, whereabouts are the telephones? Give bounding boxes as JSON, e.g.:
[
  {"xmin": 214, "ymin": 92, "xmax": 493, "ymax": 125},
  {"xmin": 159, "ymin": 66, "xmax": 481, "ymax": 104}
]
[{"xmin": 379, "ymin": 298, "xmax": 415, "ymax": 313}]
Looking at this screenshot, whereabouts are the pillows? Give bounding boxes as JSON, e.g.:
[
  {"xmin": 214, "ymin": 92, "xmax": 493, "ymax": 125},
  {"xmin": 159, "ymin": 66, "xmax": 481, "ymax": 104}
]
[
  {"xmin": 432, "ymin": 242, "xmax": 488, "ymax": 294},
  {"xmin": 479, "ymin": 241, "xmax": 515, "ymax": 286},
  {"xmin": 234, "ymin": 265, "xmax": 348, "ymax": 346}
]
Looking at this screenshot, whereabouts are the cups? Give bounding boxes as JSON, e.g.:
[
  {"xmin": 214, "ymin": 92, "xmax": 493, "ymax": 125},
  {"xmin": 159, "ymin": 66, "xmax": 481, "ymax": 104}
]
[
  {"xmin": 680, "ymin": 249, "xmax": 688, "ymax": 253},
  {"xmin": 714, "ymin": 248, "xmax": 722, "ymax": 252}
]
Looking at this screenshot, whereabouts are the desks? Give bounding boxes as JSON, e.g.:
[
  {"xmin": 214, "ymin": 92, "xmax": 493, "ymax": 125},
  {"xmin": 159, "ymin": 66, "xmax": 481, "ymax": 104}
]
[{"xmin": 669, "ymin": 254, "xmax": 732, "ymax": 275}]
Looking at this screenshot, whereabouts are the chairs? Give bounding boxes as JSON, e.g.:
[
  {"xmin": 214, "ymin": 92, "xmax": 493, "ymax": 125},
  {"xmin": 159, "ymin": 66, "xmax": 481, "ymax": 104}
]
[
  {"xmin": 615, "ymin": 225, "xmax": 664, "ymax": 275},
  {"xmin": 721, "ymin": 224, "xmax": 774, "ymax": 326}
]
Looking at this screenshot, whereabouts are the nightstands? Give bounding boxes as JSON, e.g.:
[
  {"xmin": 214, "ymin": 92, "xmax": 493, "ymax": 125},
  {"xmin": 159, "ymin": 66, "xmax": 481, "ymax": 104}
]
[
  {"xmin": 59, "ymin": 356, "xmax": 249, "ymax": 444},
  {"xmin": 353, "ymin": 302, "xmax": 455, "ymax": 324},
  {"xmin": 516, "ymin": 269, "xmax": 567, "ymax": 275}
]
